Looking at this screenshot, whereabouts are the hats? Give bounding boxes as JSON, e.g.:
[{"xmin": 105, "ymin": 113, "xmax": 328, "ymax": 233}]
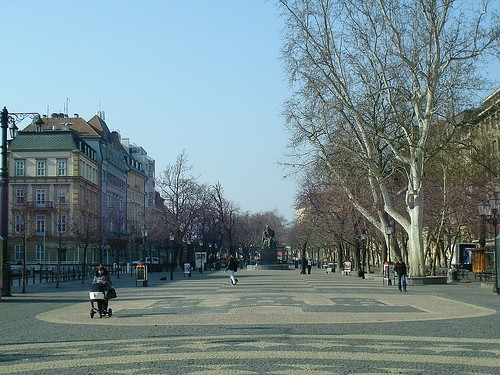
[{"xmin": 98, "ymin": 264, "xmax": 104, "ymax": 270}]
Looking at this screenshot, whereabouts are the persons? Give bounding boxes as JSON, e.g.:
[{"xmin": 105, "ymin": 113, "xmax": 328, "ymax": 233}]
[
  {"xmin": 394, "ymin": 258, "xmax": 407, "ymax": 292},
  {"xmin": 262, "ymin": 225, "xmax": 276, "ymax": 248},
  {"xmin": 224, "ymin": 255, "xmax": 238, "ymax": 285},
  {"xmin": 92, "ymin": 265, "xmax": 113, "ymax": 316},
  {"xmin": 299, "ymin": 256, "xmax": 312, "ymax": 274}
]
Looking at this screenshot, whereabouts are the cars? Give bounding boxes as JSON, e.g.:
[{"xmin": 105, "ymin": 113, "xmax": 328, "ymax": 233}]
[{"xmin": 131, "ymin": 256, "xmax": 163, "ymax": 265}]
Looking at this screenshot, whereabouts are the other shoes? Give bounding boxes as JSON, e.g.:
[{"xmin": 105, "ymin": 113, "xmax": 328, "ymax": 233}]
[
  {"xmin": 403, "ymin": 289, "xmax": 407, "ymax": 292},
  {"xmin": 399, "ymin": 290, "xmax": 402, "ymax": 292}
]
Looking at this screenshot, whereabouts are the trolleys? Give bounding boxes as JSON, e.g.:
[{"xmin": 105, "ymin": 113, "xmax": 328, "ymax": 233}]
[{"xmin": 89, "ymin": 283, "xmax": 113, "ymax": 319}]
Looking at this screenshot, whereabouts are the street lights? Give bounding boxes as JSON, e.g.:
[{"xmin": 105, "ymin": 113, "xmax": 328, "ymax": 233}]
[
  {"xmin": 0, "ymin": 105, "xmax": 46, "ymax": 301},
  {"xmin": 361, "ymin": 230, "xmax": 366, "ymax": 279},
  {"xmin": 208, "ymin": 242, "xmax": 213, "ymax": 271},
  {"xmin": 187, "ymin": 237, "xmax": 192, "ymax": 277},
  {"xmin": 476, "ymin": 194, "xmax": 500, "ymax": 295},
  {"xmin": 199, "ymin": 240, "xmax": 204, "ymax": 274},
  {"xmin": 332, "ymin": 243, "xmax": 336, "ymax": 273},
  {"xmin": 384, "ymin": 223, "xmax": 393, "ymax": 286},
  {"xmin": 141, "ymin": 224, "xmax": 149, "ymax": 265},
  {"xmin": 169, "ymin": 230, "xmax": 176, "ymax": 280}
]
